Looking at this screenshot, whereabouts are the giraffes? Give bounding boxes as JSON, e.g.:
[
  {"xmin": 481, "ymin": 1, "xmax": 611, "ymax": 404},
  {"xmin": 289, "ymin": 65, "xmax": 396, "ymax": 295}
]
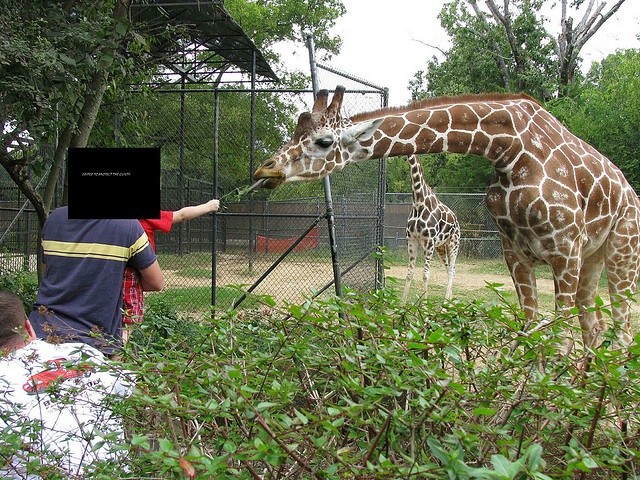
[
  {"xmin": 399, "ymin": 152, "xmax": 463, "ymax": 313},
  {"xmin": 252, "ymin": 83, "xmax": 640, "ymax": 379}
]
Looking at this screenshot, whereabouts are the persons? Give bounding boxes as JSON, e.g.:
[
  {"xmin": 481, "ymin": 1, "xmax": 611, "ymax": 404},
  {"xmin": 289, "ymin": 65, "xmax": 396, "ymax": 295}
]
[
  {"xmin": 0, "ymin": 290, "xmax": 133, "ymax": 480},
  {"xmin": 110, "ymin": 198, "xmax": 220, "ymax": 361},
  {"xmin": 29, "ymin": 205, "xmax": 163, "ymax": 358}
]
